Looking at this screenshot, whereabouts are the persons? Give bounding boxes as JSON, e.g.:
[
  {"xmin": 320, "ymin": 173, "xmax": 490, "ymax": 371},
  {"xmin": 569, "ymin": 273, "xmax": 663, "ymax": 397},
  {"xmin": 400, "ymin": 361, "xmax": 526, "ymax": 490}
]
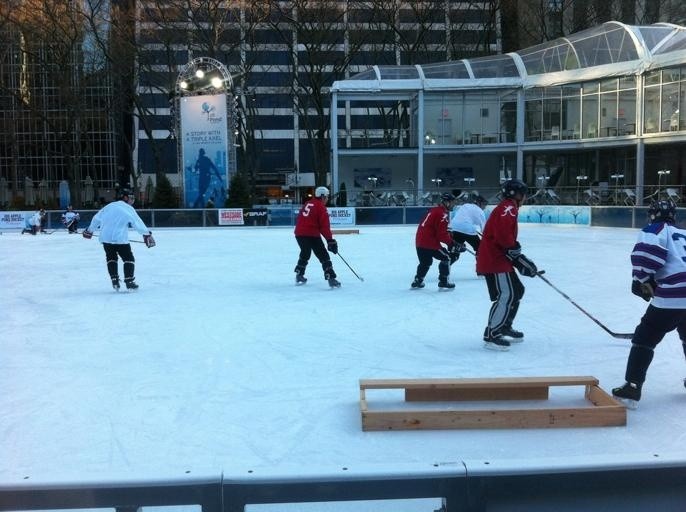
[
  {"xmin": 474, "ymin": 179, "xmax": 539, "ymax": 348},
  {"xmin": 187, "ymin": 148, "xmax": 223, "ymax": 208},
  {"xmin": 82, "ymin": 189, "xmax": 155, "ymax": 293},
  {"xmin": 206, "ymin": 195, "xmax": 216, "ymax": 208},
  {"xmin": 22, "ymin": 207, "xmax": 48, "ymax": 235},
  {"xmin": 411, "ymin": 192, "xmax": 467, "ymax": 289},
  {"xmin": 121, "ymin": 193, "xmax": 136, "ymax": 229},
  {"xmin": 59, "ymin": 204, "xmax": 80, "ymax": 233},
  {"xmin": 449, "ymin": 191, "xmax": 488, "ymax": 276},
  {"xmin": 294, "ymin": 186, "xmax": 343, "ymax": 289},
  {"xmin": 611, "ymin": 200, "xmax": 686, "ymax": 401}
]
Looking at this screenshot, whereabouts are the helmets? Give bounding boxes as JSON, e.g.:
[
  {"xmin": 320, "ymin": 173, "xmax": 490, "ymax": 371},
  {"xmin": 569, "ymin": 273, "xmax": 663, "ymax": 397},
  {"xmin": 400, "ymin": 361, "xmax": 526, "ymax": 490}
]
[
  {"xmin": 440, "ymin": 179, "xmax": 528, "ymax": 212},
  {"xmin": 315, "ymin": 187, "xmax": 329, "ymax": 197},
  {"xmin": 117, "ymin": 189, "xmax": 136, "ymax": 202},
  {"xmin": 647, "ymin": 200, "xmax": 676, "ymax": 226}
]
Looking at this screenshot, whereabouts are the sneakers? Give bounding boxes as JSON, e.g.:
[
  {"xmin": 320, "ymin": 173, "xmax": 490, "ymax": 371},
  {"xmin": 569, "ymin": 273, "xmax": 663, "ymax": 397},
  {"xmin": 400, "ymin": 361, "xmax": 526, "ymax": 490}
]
[
  {"xmin": 112, "ymin": 279, "xmax": 120, "ymax": 289},
  {"xmin": 612, "ymin": 382, "xmax": 641, "ymax": 402},
  {"xmin": 438, "ymin": 281, "xmax": 455, "ymax": 288},
  {"xmin": 483, "ymin": 332, "xmax": 511, "ymax": 346},
  {"xmin": 502, "ymin": 326, "xmax": 523, "ymax": 338},
  {"xmin": 126, "ymin": 282, "xmax": 139, "ymax": 288},
  {"xmin": 328, "ymin": 279, "xmax": 340, "ymax": 286},
  {"xmin": 296, "ymin": 275, "xmax": 306, "ymax": 282},
  {"xmin": 412, "ymin": 282, "xmax": 425, "ymax": 287}
]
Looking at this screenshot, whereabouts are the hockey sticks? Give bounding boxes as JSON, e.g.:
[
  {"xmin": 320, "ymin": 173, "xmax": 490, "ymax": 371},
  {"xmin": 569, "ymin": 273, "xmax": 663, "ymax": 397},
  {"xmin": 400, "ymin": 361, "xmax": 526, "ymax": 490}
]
[
  {"xmin": 337, "ymin": 251, "xmax": 371, "ymax": 282},
  {"xmin": 532, "ymin": 269, "xmax": 637, "ymax": 340},
  {"xmin": 72, "ymin": 230, "xmax": 150, "ymax": 245}
]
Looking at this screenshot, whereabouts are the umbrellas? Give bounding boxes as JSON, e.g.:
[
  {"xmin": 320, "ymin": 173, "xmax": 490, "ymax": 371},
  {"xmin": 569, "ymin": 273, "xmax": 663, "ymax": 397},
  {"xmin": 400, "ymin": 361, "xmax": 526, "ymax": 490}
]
[{"xmin": 0, "ymin": 173, "xmax": 154, "ymax": 210}]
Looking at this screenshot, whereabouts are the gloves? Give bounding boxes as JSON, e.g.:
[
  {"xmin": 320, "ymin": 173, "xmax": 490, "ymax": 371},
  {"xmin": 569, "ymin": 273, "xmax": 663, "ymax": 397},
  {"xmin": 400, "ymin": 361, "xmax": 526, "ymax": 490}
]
[
  {"xmin": 143, "ymin": 231, "xmax": 155, "ymax": 248},
  {"xmin": 83, "ymin": 229, "xmax": 92, "ymax": 239},
  {"xmin": 514, "ymin": 254, "xmax": 536, "ymax": 276},
  {"xmin": 328, "ymin": 239, "xmax": 338, "ymax": 254},
  {"xmin": 450, "ymin": 241, "xmax": 466, "ymax": 252},
  {"xmin": 632, "ymin": 280, "xmax": 656, "ymax": 301}
]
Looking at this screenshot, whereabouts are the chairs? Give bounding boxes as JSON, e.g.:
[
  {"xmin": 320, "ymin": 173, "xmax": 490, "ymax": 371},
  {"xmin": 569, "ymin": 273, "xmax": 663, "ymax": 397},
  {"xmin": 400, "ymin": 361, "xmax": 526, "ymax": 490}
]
[
  {"xmin": 550, "ymin": 123, "xmax": 600, "ymax": 140},
  {"xmin": 645, "ymin": 111, "xmax": 679, "ymax": 132}
]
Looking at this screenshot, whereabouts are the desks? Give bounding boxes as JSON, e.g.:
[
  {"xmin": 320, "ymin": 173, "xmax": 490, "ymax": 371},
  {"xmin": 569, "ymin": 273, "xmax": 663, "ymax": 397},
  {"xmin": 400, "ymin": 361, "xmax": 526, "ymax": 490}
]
[
  {"xmin": 600, "ymin": 122, "xmax": 635, "ymax": 136},
  {"xmin": 536, "ymin": 129, "xmax": 550, "ymax": 139}
]
